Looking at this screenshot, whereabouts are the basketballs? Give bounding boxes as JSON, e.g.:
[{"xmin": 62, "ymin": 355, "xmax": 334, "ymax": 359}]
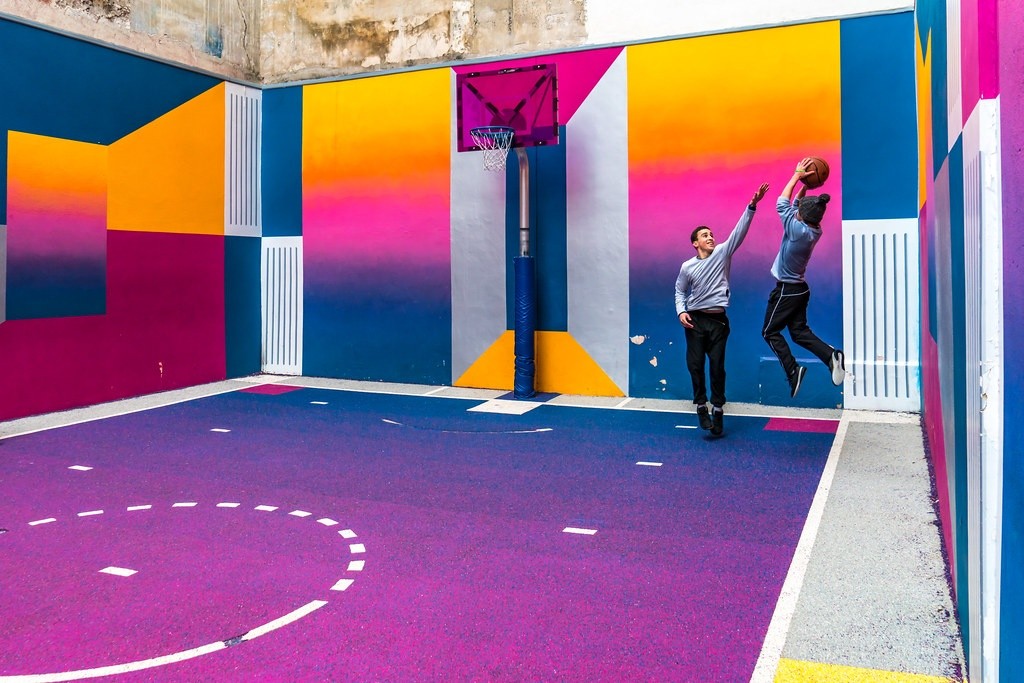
[{"xmin": 799, "ymin": 157, "xmax": 830, "ymax": 187}]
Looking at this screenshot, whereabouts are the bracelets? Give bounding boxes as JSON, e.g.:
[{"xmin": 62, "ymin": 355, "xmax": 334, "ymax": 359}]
[
  {"xmin": 795, "ymin": 170, "xmax": 805, "ymax": 172},
  {"xmin": 749, "ymin": 204, "xmax": 756, "ymax": 211}
]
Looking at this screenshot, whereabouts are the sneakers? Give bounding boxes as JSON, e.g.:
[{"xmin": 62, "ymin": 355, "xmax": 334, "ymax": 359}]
[
  {"xmin": 828, "ymin": 349, "xmax": 844, "ymax": 386},
  {"xmin": 785, "ymin": 366, "xmax": 807, "ymax": 398},
  {"xmin": 710, "ymin": 407, "xmax": 723, "ymax": 435},
  {"xmin": 697, "ymin": 406, "xmax": 714, "ymax": 430}
]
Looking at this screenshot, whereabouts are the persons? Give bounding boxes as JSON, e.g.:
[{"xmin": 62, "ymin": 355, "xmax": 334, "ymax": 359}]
[
  {"xmin": 761, "ymin": 158, "xmax": 845, "ymax": 397},
  {"xmin": 675, "ymin": 182, "xmax": 769, "ymax": 435}
]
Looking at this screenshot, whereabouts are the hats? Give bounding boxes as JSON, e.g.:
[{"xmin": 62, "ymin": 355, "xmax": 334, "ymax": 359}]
[{"xmin": 799, "ymin": 193, "xmax": 830, "ymax": 224}]
